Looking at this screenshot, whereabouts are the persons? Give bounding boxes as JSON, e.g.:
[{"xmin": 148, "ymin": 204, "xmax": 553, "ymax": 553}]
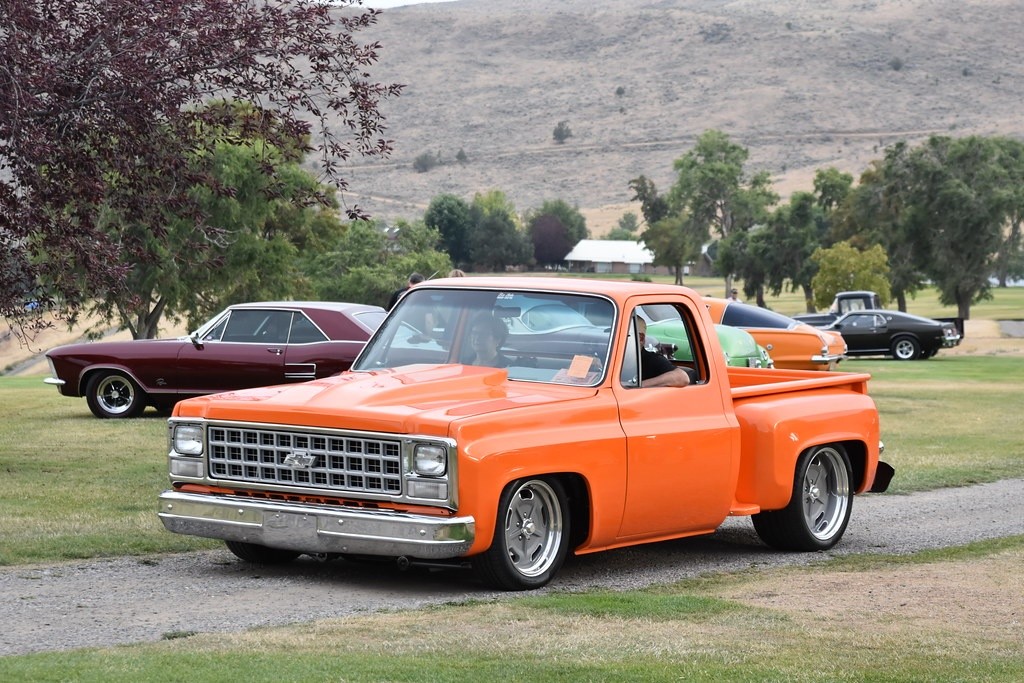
[
  {"xmin": 396, "ymin": 270, "xmax": 471, "ymax": 346},
  {"xmin": 463, "ymin": 313, "xmax": 512, "ymax": 369},
  {"xmin": 588, "ymin": 314, "xmax": 690, "ymax": 388},
  {"xmin": 727, "ymin": 288, "xmax": 742, "ymax": 305}
]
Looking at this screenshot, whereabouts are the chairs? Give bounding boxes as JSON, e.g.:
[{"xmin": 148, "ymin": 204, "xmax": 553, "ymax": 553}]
[{"xmin": 267, "ymin": 315, "xmax": 291, "ymax": 343}]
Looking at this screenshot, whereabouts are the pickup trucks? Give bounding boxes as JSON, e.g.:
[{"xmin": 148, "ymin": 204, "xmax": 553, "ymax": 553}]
[{"xmin": 158, "ymin": 277, "xmax": 896, "ymax": 592}]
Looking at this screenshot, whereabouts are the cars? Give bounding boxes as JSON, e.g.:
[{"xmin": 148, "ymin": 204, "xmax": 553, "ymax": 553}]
[{"xmin": 790, "ymin": 290, "xmax": 892, "ymax": 327}]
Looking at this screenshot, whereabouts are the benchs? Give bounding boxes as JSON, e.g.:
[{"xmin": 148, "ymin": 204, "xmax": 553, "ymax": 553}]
[{"xmin": 498, "ymin": 349, "xmax": 697, "ymax": 385}]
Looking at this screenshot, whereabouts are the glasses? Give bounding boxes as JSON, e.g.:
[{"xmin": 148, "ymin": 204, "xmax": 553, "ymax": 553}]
[{"xmin": 470, "ymin": 330, "xmax": 492, "ymax": 338}]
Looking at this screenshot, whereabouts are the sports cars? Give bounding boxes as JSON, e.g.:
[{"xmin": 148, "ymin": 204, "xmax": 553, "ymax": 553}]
[
  {"xmin": 818, "ymin": 308, "xmax": 961, "ymax": 361},
  {"xmin": 45, "ymin": 301, "xmax": 539, "ymax": 419},
  {"xmin": 436, "ymin": 293, "xmax": 849, "ymax": 376}
]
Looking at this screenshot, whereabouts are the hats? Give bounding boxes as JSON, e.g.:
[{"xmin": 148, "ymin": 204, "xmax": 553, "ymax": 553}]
[{"xmin": 604, "ymin": 314, "xmax": 646, "ymax": 335}]
